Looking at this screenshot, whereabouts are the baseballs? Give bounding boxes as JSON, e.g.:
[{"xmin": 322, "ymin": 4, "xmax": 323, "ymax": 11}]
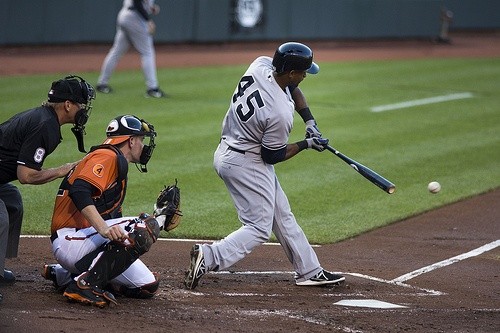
[{"xmin": 428, "ymin": 182, "xmax": 441, "ymax": 194}]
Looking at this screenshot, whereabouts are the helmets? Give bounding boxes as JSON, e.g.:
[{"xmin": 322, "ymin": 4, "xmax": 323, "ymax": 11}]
[
  {"xmin": 103, "ymin": 114, "xmax": 146, "ymax": 145},
  {"xmin": 272, "ymin": 41, "xmax": 319, "ymax": 75}
]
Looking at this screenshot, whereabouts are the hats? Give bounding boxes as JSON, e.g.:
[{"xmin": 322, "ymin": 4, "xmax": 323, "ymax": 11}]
[{"xmin": 47, "ymin": 78, "xmax": 87, "ymax": 105}]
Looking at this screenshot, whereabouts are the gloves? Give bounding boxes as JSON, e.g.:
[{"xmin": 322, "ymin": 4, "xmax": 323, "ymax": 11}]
[
  {"xmin": 305, "ymin": 120, "xmax": 321, "ymax": 139},
  {"xmin": 305, "ymin": 134, "xmax": 329, "ymax": 151}
]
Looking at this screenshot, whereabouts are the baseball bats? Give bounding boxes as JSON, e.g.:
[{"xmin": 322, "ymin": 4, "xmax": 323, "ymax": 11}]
[{"xmin": 324, "ymin": 143, "xmax": 396, "ymax": 194}]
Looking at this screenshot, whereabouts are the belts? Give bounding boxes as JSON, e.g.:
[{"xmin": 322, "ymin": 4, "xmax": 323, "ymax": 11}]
[
  {"xmin": 227, "ymin": 146, "xmax": 246, "ymax": 155},
  {"xmin": 50, "ymin": 228, "xmax": 81, "ymax": 244}
]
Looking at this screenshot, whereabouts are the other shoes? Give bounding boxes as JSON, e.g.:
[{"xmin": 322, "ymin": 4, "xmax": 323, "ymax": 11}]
[
  {"xmin": 145, "ymin": 88, "xmax": 164, "ymax": 98},
  {"xmin": 0, "ymin": 269, "xmax": 16, "ymax": 285},
  {"xmin": 96, "ymin": 83, "xmax": 111, "ymax": 93}
]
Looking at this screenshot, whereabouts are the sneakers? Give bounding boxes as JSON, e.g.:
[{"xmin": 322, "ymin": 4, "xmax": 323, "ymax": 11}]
[
  {"xmin": 63, "ymin": 281, "xmax": 109, "ymax": 307},
  {"xmin": 42, "ymin": 264, "xmax": 57, "ymax": 282},
  {"xmin": 182, "ymin": 243, "xmax": 208, "ymax": 291},
  {"xmin": 295, "ymin": 268, "xmax": 345, "ymax": 286}
]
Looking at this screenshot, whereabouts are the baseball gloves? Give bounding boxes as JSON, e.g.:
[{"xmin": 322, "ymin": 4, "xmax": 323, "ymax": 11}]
[{"xmin": 157, "ymin": 185, "xmax": 181, "ymax": 231}]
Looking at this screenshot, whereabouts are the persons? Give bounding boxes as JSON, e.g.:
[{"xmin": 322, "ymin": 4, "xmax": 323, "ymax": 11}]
[
  {"xmin": 42, "ymin": 114, "xmax": 168, "ymax": 306},
  {"xmin": 96, "ymin": 0, "xmax": 165, "ymax": 98},
  {"xmin": 184, "ymin": 42, "xmax": 345, "ymax": 290},
  {"xmin": 0, "ymin": 75, "xmax": 95, "ymax": 291}
]
